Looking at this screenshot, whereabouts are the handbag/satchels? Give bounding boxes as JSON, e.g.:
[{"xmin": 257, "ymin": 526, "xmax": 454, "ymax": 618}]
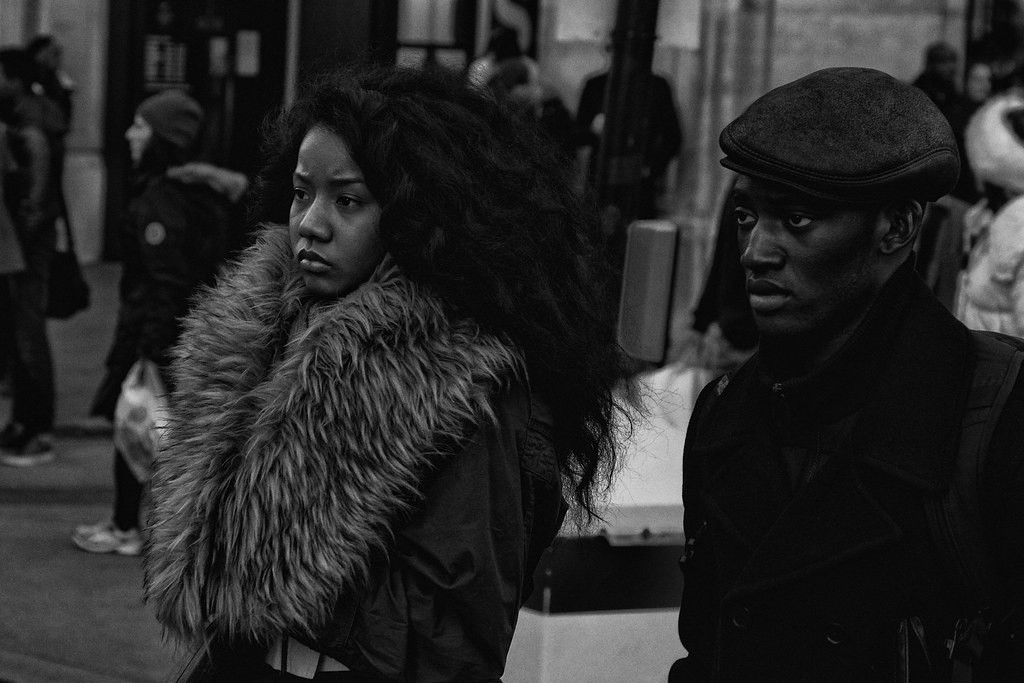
[
  {"xmin": 112, "ymin": 358, "xmax": 172, "ymax": 484},
  {"xmin": 31, "ymin": 249, "xmax": 92, "ymax": 319}
]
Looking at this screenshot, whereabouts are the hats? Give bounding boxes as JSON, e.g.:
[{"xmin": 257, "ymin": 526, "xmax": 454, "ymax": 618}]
[
  {"xmin": 719, "ymin": 67, "xmax": 961, "ymax": 203},
  {"xmin": 137, "ymin": 88, "xmax": 203, "ymax": 149}
]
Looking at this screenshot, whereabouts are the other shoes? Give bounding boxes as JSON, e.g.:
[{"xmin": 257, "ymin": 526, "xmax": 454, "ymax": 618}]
[
  {"xmin": 0, "ymin": 418, "xmax": 55, "ymax": 466},
  {"xmin": 69, "ymin": 516, "xmax": 143, "ymax": 555}
]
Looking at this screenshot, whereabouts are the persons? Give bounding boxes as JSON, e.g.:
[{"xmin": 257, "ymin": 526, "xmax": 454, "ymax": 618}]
[
  {"xmin": 136, "ymin": 57, "xmax": 647, "ymax": 682},
  {"xmin": 458, "ymin": 14, "xmax": 685, "ymax": 322},
  {"xmin": 670, "ymin": 30, "xmax": 1024, "ymax": 682},
  {"xmin": 71, "ymin": 86, "xmax": 255, "ymax": 560},
  {"xmin": 0, "ymin": 35, "xmax": 90, "ymax": 466}
]
